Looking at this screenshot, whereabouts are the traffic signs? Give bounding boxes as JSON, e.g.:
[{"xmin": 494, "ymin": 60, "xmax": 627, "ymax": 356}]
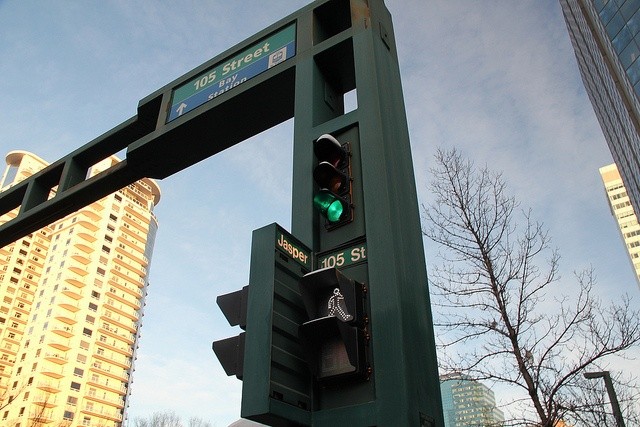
[
  {"xmin": 164, "ymin": 18, "xmax": 298, "ymax": 125},
  {"xmin": 317, "ymin": 241, "xmax": 368, "ymax": 269}
]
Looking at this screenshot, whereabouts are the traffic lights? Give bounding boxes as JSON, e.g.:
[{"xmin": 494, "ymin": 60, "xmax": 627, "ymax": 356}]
[
  {"xmin": 313, "ymin": 133, "xmax": 357, "ymax": 232},
  {"xmin": 212, "ymin": 283, "xmax": 249, "ymax": 380},
  {"xmin": 299, "ymin": 267, "xmax": 373, "ymax": 393}
]
